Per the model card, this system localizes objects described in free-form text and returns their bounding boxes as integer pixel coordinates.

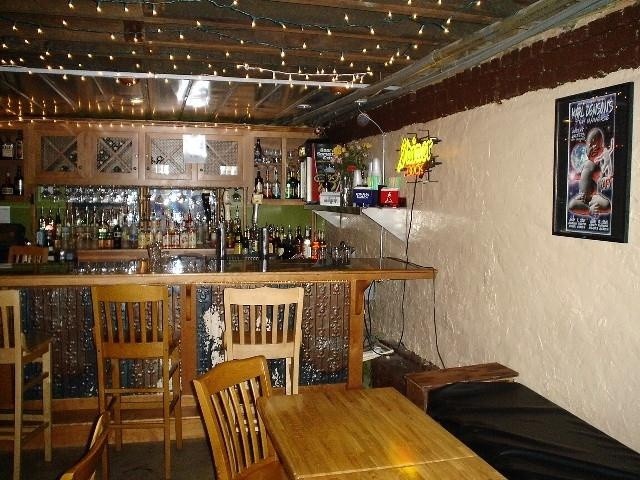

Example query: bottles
[225,210,351,269]
[322,174,332,193]
[339,168,353,208]
[0,131,23,196]
[97,137,125,173]
[48,240,77,273]
[37,206,219,249]
[57,150,77,172]
[254,138,301,199]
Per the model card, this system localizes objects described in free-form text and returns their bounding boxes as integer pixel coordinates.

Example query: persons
[569,126,611,217]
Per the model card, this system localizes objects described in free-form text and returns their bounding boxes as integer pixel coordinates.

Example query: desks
[256,386,508,480]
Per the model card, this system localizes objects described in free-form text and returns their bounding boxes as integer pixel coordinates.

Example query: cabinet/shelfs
[250,127,320,207]
[0,128,29,204]
[139,131,244,188]
[31,125,139,188]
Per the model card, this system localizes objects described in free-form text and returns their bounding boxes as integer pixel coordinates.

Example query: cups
[353,158,382,190]
[78,255,219,275]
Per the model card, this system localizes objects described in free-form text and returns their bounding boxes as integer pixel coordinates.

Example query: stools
[405,362,518,413]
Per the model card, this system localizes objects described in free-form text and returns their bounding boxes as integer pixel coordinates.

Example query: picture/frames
[552,80,635,244]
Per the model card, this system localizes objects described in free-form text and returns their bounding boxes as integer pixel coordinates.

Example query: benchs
[424,382,623,479]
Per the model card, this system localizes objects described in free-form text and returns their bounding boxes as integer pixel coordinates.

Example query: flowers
[330,138,373,171]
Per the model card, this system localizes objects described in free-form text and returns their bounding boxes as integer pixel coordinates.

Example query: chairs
[8,245,49,265]
[91,283,183,479]
[0,289,54,479]
[192,356,291,480]
[223,286,305,434]
[59,411,111,480]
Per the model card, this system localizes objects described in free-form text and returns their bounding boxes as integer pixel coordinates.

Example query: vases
[353,171,363,189]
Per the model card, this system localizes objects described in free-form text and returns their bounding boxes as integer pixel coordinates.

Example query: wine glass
[40,184,217,205]
